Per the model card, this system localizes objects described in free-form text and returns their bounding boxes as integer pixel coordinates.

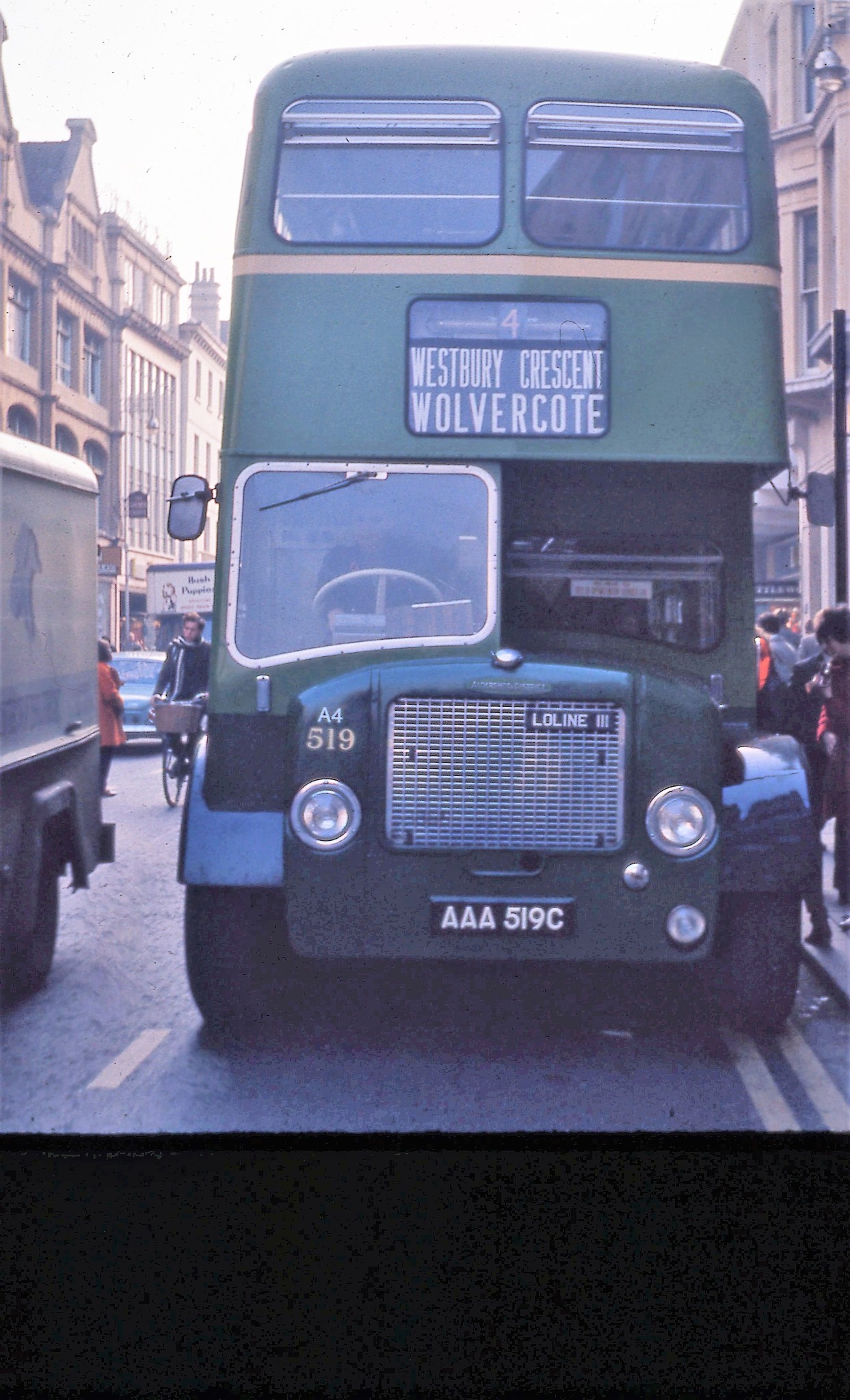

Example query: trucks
[146,562,216,653]
[0,432,116,1013]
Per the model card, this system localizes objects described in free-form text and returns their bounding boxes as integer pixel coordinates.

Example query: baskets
[154,702,201,734]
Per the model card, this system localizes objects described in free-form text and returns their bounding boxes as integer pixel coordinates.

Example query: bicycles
[153,693,206,806]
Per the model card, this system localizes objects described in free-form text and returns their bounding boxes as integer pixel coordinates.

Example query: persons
[154,612,212,776]
[117,615,178,651]
[756,601,850,943]
[95,639,124,795]
[314,500,465,626]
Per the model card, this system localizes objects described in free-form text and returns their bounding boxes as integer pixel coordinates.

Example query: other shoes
[838,916,850,929]
[805,927,831,946]
[100,789,116,796]
[169,758,185,777]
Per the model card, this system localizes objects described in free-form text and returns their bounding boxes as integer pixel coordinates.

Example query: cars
[108,651,168,747]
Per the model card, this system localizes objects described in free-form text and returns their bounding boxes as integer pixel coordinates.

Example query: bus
[162,46,812,1041]
